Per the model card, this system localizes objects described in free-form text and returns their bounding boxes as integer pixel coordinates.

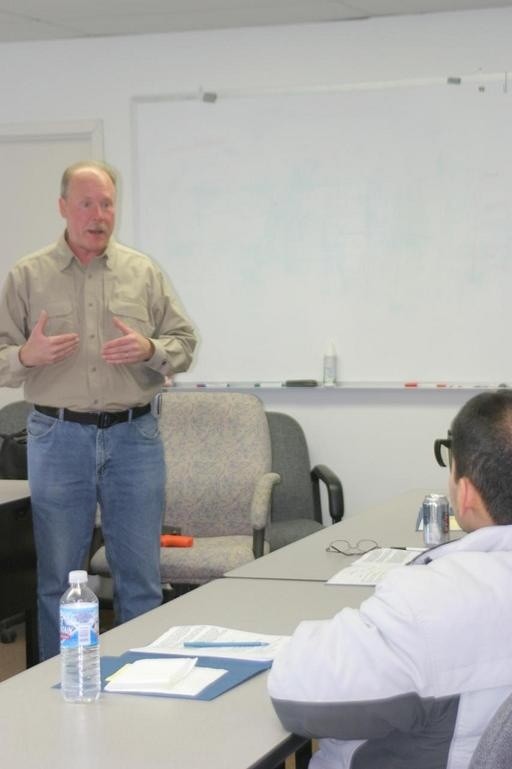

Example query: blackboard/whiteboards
[117,72,512,391]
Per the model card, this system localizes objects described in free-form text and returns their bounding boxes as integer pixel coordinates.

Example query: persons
[1,160,197,663]
[265,386,512,768]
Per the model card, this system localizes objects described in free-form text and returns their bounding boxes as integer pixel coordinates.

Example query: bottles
[59,568,104,702]
[323,341,336,388]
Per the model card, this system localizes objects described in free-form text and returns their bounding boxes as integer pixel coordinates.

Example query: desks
[225,487,471,580]
[2,576,382,768]
[0,479,34,509]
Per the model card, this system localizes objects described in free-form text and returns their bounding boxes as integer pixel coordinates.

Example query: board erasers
[285,379,318,387]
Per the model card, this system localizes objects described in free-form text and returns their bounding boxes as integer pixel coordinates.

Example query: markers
[404,382,507,388]
[172,382,262,388]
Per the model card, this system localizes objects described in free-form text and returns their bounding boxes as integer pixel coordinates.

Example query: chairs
[1,399,44,638]
[87,389,284,604]
[264,412,344,551]
[470,693,512,769]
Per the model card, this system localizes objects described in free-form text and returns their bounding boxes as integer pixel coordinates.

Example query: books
[325,547,422,588]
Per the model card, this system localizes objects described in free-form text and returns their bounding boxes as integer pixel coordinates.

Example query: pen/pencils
[184,641,268,648]
[391,546,430,551]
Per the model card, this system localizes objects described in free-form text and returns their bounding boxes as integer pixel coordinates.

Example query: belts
[32,402,153,430]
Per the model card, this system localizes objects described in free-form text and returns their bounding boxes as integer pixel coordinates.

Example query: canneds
[423,493,450,547]
